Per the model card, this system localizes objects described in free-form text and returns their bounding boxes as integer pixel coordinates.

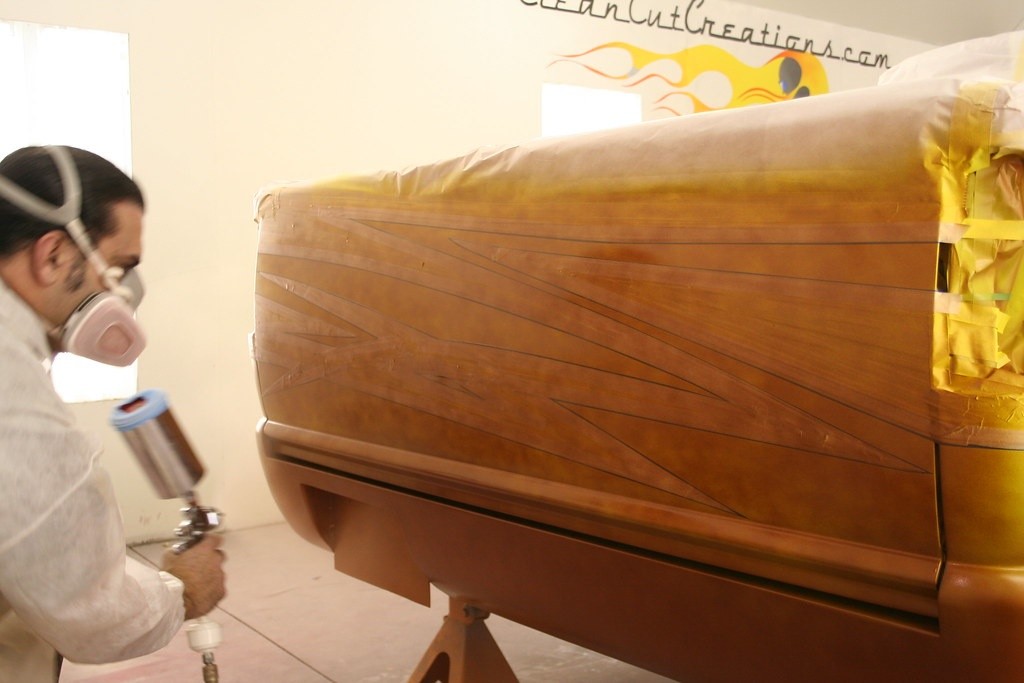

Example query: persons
[0,145,226,683]
